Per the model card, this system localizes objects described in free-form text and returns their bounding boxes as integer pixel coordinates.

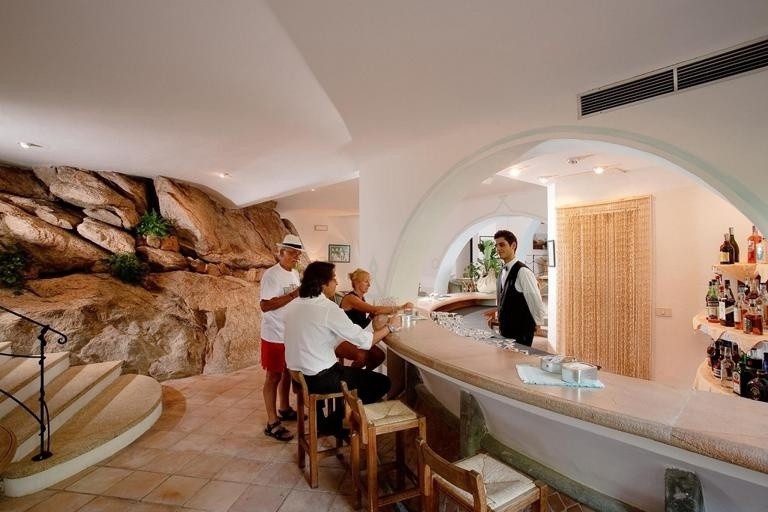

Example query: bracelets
[392,305,395,313]
[384,322,396,333]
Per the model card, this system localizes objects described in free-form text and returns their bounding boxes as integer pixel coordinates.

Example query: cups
[400,315,410,328]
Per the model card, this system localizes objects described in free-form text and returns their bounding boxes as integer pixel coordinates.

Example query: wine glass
[424,287,438,307]
[431,312,464,335]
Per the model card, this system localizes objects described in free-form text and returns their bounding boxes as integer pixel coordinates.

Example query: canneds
[402,314,411,328]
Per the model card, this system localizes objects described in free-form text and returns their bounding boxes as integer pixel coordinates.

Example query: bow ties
[499,264,508,273]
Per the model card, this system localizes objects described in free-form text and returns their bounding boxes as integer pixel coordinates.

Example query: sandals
[264,421,295,442]
[277,406,308,421]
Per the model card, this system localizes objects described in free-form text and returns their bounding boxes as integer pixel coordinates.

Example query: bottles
[746,226,767,264]
[704,274,767,333]
[718,232,732,264]
[724,226,738,262]
[704,338,767,402]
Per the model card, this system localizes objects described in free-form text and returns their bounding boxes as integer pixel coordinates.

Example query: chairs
[289,369,358,489]
[340,381,427,512]
[415,436,549,512]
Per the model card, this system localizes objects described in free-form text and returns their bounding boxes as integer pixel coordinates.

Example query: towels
[516,363,605,389]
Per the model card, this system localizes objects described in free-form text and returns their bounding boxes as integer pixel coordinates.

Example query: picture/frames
[328,244,351,263]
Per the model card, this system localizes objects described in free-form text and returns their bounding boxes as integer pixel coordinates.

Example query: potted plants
[463,239,504,293]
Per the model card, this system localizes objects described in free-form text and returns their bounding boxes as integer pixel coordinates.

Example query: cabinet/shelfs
[693,262,768,396]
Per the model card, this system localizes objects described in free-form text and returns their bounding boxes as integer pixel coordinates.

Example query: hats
[275,234,305,253]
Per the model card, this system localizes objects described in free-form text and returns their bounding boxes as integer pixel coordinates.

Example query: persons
[492,229,545,348]
[255,234,310,444]
[333,268,414,370]
[281,261,400,440]
